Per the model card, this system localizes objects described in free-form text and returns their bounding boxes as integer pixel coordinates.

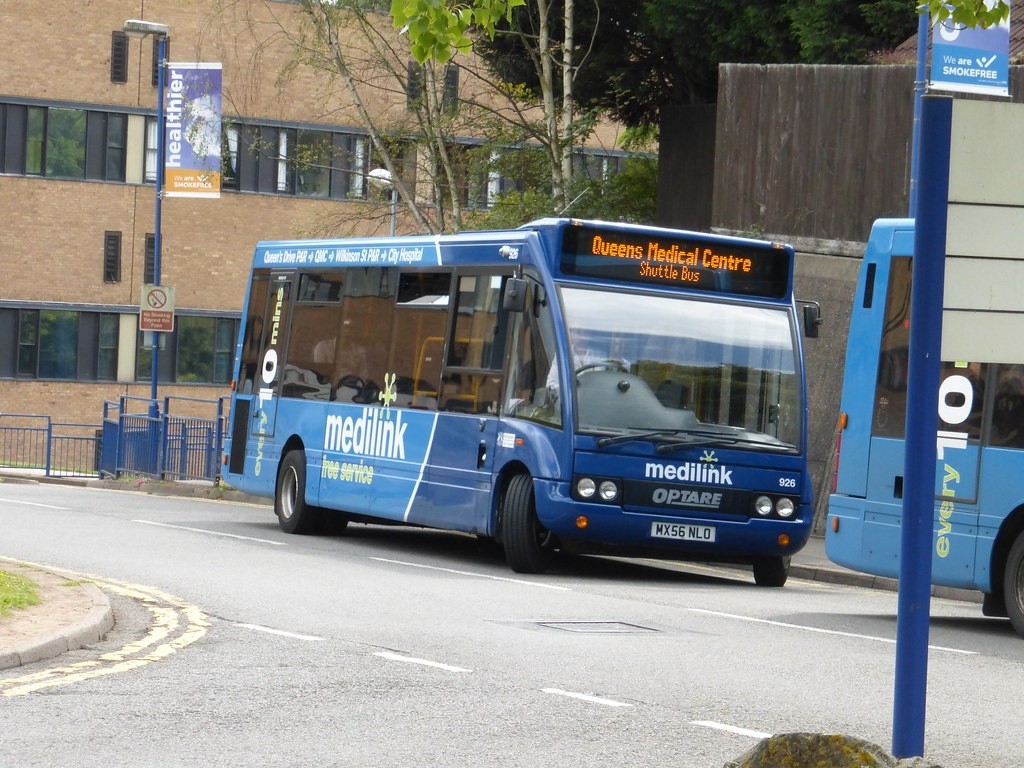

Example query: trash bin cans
[94,428,154,473]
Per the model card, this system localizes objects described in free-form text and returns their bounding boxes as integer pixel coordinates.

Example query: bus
[824,216,1024,639]
[222,215,817,588]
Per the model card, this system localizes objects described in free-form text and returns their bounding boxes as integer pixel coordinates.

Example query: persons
[313,318,369,381]
[545,314,607,389]
